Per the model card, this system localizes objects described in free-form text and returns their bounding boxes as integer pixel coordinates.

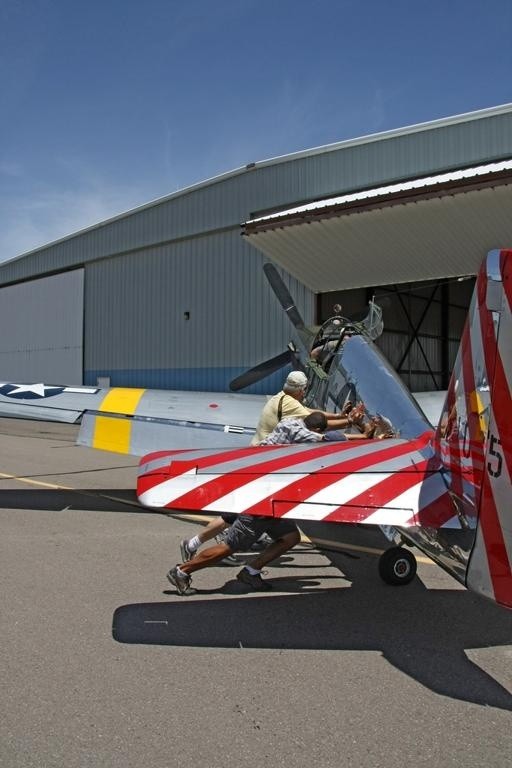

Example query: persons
[177,369,366,567]
[308,323,351,364]
[164,411,396,596]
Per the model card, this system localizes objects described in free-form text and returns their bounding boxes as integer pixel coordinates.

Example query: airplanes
[131,242,512,614]
[0,257,454,593]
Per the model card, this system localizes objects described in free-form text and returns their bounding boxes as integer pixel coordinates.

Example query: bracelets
[346,417,353,426]
[334,412,341,419]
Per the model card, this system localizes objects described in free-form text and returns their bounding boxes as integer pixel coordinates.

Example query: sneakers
[236,567,273,592]
[178,539,197,566]
[214,526,271,565]
[165,563,197,596]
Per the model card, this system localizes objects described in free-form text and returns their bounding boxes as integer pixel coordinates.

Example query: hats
[339,326,355,336]
[286,370,312,386]
